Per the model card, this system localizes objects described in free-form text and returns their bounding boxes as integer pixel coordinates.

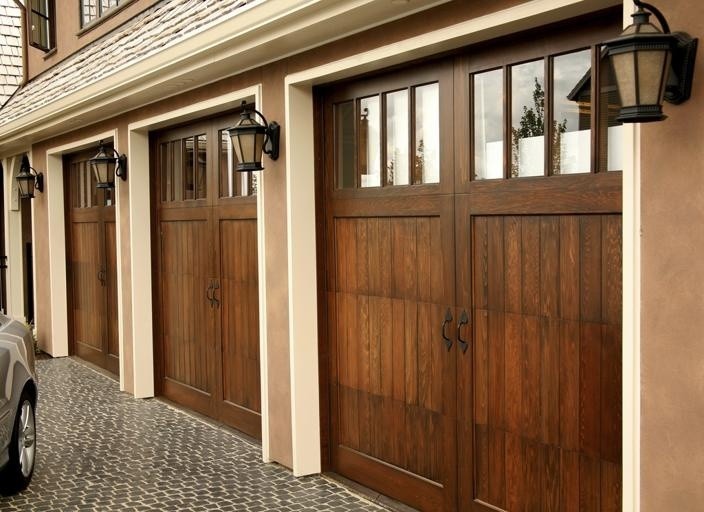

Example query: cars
[0,312,39,489]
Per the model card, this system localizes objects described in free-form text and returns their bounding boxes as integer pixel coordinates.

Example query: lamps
[227,101,281,176]
[601,1,698,127]
[87,140,127,189]
[15,154,44,201]
